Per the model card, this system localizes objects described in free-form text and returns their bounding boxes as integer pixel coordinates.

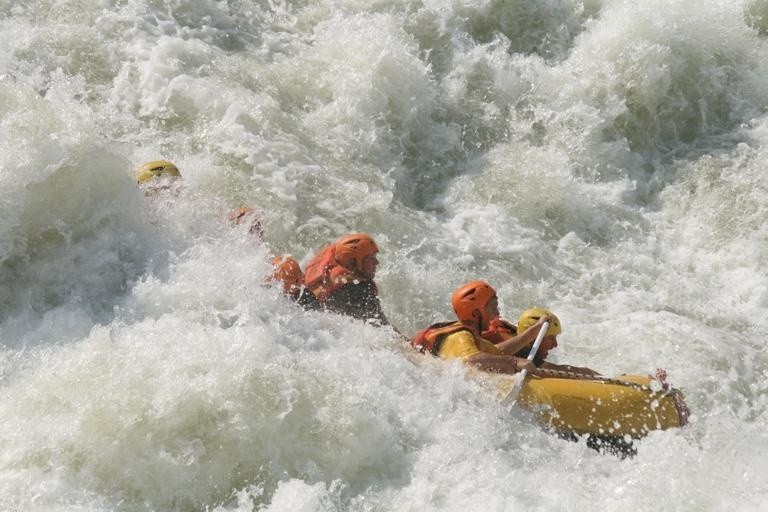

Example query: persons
[410,281,552,376]
[481,308,603,377]
[272,258,321,312]
[309,232,389,324]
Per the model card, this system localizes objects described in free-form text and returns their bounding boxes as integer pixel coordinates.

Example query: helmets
[227,207,263,235]
[272,257,305,293]
[452,283,497,327]
[515,309,560,338]
[335,235,378,274]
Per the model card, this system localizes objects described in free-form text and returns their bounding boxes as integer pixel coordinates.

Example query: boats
[458,362,694,461]
